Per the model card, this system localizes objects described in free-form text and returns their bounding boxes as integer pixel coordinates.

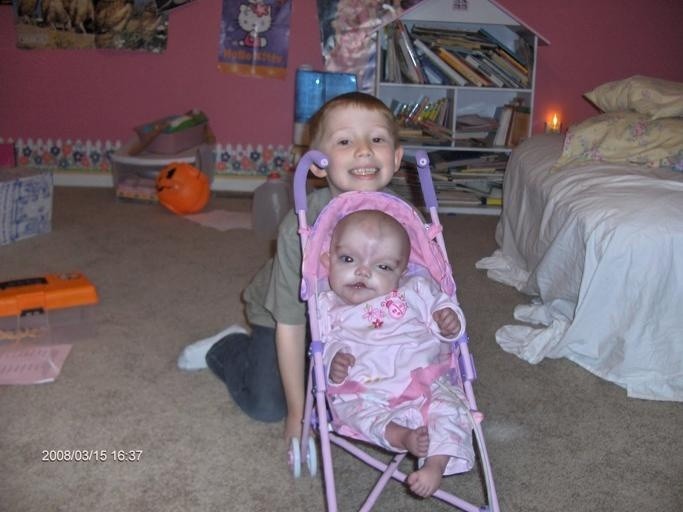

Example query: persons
[317,209,476,500]
[177,90,427,446]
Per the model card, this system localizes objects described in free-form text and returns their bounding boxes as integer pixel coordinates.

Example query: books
[385,19,534,89]
[391,149,509,207]
[389,94,452,147]
[455,96,531,148]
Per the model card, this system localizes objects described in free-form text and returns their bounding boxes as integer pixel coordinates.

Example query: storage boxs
[106,149,196,205]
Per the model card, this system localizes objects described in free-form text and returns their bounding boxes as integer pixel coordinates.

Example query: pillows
[583,74,683,120]
[553,111,683,173]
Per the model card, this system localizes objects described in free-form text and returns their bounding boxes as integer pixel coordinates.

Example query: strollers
[285,147,501,512]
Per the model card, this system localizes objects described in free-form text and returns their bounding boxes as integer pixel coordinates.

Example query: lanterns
[155,161,211,215]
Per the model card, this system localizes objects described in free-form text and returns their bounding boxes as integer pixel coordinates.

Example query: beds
[475,131,683,403]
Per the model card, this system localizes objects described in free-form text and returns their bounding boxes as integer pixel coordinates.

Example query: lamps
[552,114,558,132]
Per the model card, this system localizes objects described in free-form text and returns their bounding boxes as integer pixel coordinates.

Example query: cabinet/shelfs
[365,0,551,216]
